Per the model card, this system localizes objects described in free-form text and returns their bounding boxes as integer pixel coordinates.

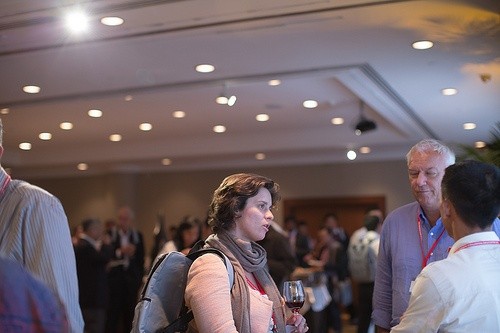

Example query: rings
[304,324,309,330]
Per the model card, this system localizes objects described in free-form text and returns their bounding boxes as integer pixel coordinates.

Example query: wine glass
[283,281,306,326]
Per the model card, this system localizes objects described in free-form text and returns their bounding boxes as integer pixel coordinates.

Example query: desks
[290,269,327,333]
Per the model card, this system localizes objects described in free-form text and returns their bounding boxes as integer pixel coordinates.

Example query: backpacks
[130,240,234,333]
[348,239,378,283]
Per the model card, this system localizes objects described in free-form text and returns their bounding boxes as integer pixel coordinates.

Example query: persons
[390,159,500,333]
[0,119,211,333]
[185,174,310,333]
[371,139,500,333]
[256,208,385,333]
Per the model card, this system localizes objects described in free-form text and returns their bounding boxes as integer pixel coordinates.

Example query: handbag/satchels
[335,281,353,306]
[310,284,332,312]
